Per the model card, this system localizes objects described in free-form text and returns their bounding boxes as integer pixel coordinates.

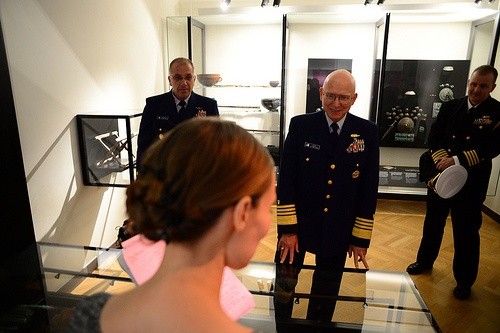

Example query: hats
[428,164,468,199]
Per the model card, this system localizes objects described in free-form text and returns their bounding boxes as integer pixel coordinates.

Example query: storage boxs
[76,108,143,188]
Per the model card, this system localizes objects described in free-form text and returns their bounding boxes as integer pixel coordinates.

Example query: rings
[281,247,284,250]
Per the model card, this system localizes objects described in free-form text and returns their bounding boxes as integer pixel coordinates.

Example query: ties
[468,106,476,115]
[330,122,340,143]
[178,100,186,114]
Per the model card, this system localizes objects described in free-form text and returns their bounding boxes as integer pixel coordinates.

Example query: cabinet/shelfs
[197,85,281,135]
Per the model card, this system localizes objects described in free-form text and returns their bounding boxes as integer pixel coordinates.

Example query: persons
[406,65,500,300]
[48,116,277,333]
[272,68,380,333]
[306,78,322,113]
[135,57,220,177]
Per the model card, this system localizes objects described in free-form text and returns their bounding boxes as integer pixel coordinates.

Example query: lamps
[260,0,269,7]
[220,0,232,11]
[377,0,385,6]
[273,0,280,7]
[488,0,496,5]
[474,0,482,6]
[363,0,374,6]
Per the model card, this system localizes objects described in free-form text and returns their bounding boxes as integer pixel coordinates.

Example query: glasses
[170,74,196,81]
[322,87,355,104]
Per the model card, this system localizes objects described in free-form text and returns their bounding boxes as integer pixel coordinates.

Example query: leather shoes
[453,286,471,300]
[407,262,428,275]
